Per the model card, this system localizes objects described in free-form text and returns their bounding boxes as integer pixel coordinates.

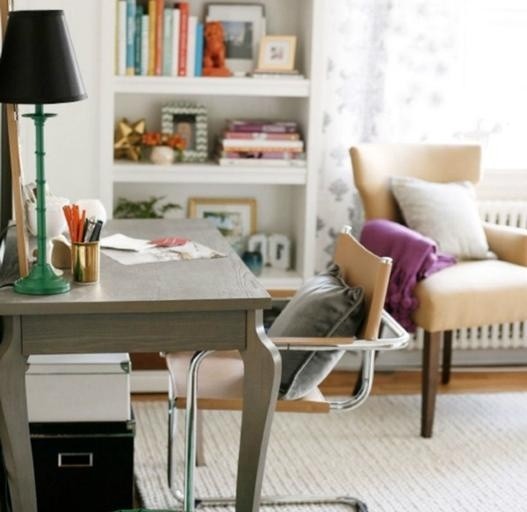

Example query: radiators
[400,205,526,354]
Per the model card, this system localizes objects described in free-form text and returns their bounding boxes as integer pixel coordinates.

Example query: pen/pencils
[101,247,139,252]
[63,203,102,242]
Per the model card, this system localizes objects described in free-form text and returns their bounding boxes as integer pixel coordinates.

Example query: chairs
[349,143,527,437]
[165,225,409,512]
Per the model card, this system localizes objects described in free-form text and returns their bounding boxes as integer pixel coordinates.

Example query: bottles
[75,198,107,230]
[25,196,71,239]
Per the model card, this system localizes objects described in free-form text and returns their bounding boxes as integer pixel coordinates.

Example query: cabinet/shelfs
[103,0,318,298]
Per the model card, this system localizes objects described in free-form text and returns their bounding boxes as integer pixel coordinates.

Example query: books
[213,117,305,165]
[114,1,207,78]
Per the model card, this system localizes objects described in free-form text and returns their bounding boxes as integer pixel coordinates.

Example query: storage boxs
[26,353,132,424]
[31,420,133,512]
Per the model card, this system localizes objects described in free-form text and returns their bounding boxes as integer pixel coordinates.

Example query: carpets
[134,391,527,512]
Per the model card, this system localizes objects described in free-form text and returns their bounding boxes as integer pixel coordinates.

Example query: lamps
[1,10,90,298]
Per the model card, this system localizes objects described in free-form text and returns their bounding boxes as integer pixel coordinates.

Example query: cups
[71,242,100,284]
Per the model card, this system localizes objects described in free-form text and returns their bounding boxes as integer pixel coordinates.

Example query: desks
[0,218,283,512]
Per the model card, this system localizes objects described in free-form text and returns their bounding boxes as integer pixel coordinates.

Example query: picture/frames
[186,198,257,252]
[257,34,298,71]
[161,100,210,163]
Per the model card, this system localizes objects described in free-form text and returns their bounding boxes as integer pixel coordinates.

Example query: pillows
[390,170,497,261]
[268,263,366,401]
